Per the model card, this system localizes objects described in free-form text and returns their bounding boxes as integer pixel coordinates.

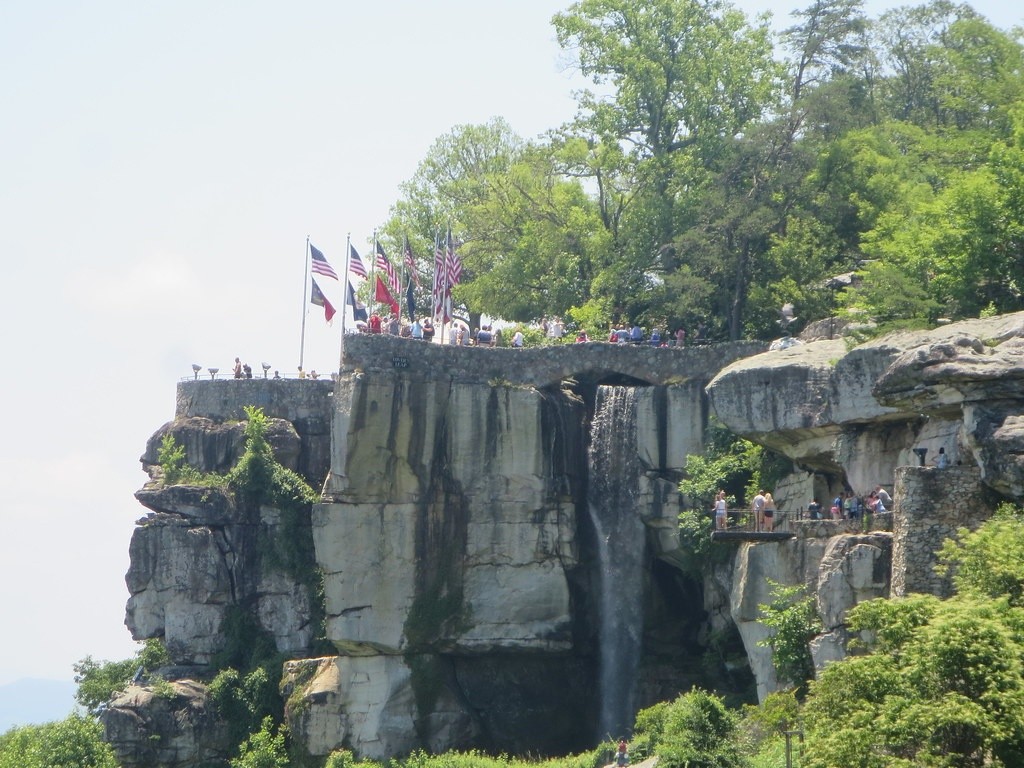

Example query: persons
[694,324,708,344]
[808,485,891,519]
[529,316,565,340]
[273,371,281,379]
[233,357,252,379]
[298,366,305,378]
[614,742,629,768]
[578,330,592,342]
[311,371,317,378]
[450,323,503,347]
[609,324,684,347]
[510,328,523,347]
[931,447,950,468]
[357,311,435,340]
[711,492,727,530]
[751,490,774,532]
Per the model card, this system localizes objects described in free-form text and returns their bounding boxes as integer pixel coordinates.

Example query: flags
[310,244,340,281]
[346,228,461,324]
[311,276,335,322]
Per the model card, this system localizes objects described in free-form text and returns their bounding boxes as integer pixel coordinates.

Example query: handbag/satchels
[610,335,618,341]
[817,512,823,519]
[831,507,839,514]
